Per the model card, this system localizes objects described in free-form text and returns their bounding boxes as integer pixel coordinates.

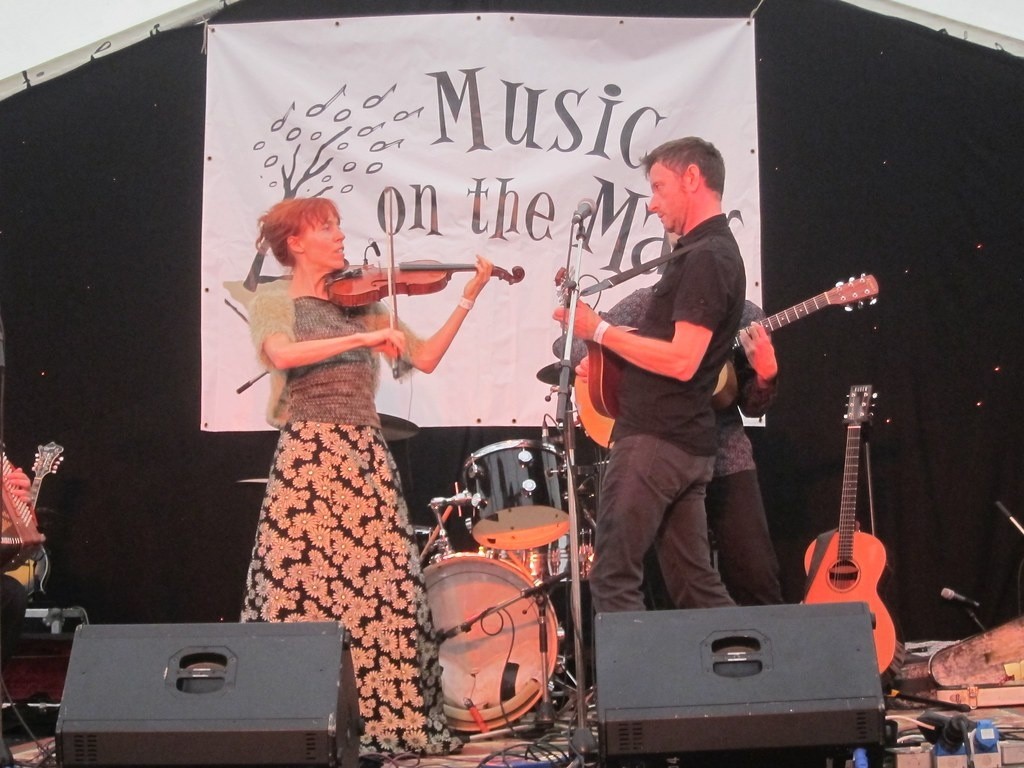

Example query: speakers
[54,621,367,767]
[589,600,885,768]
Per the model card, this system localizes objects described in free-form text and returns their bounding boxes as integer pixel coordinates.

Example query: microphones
[541,420,550,444]
[571,197,597,224]
[940,588,980,607]
[937,714,967,754]
[362,242,381,266]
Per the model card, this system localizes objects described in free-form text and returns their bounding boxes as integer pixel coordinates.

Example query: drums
[463,438,569,550]
[478,464,596,583]
[422,553,558,731]
[415,526,452,570]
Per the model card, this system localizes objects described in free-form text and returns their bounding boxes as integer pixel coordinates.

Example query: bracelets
[458,296,475,311]
[593,319,611,345]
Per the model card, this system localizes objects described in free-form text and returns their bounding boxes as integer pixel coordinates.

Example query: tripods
[433,568,596,744]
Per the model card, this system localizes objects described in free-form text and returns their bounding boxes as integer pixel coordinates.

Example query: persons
[552,137,747,617]
[237,197,494,759]
[587,285,784,611]
[0,441,33,675]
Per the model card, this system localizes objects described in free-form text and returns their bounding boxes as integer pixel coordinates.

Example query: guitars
[574,273,879,451]
[555,265,639,420]
[799,384,906,687]
[4,441,63,598]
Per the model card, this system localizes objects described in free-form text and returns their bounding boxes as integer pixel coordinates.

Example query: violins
[327,259,526,307]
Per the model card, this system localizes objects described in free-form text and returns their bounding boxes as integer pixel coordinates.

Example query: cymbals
[535,360,581,390]
[376,412,420,441]
[235,479,269,484]
